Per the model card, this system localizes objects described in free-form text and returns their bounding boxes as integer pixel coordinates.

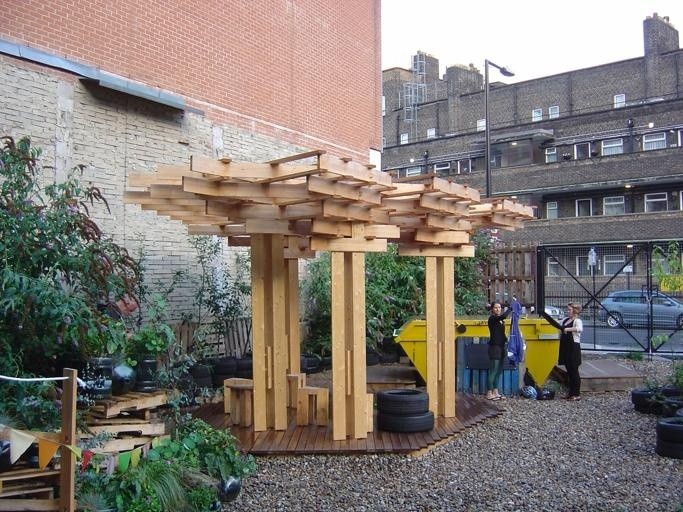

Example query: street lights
[421,149,430,175]
[482,57,514,198]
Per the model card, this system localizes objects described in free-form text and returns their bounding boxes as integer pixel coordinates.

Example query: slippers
[486,396,507,400]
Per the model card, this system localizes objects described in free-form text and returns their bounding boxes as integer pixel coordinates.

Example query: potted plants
[124,325,163,394]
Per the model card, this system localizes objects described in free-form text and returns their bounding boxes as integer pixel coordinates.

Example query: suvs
[598,290,682,329]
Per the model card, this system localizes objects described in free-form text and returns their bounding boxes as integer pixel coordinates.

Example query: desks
[89,386,180,421]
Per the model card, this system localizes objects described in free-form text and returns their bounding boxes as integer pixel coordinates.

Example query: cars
[476,291,564,321]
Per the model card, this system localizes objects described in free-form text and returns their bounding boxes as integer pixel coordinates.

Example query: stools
[223,373,331,433]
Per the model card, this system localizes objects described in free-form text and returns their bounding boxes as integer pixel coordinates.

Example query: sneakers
[566,395,581,401]
[560,393,574,399]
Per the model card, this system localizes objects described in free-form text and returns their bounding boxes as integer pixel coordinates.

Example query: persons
[103,294,137,342]
[558,302,583,401]
[486,295,517,401]
